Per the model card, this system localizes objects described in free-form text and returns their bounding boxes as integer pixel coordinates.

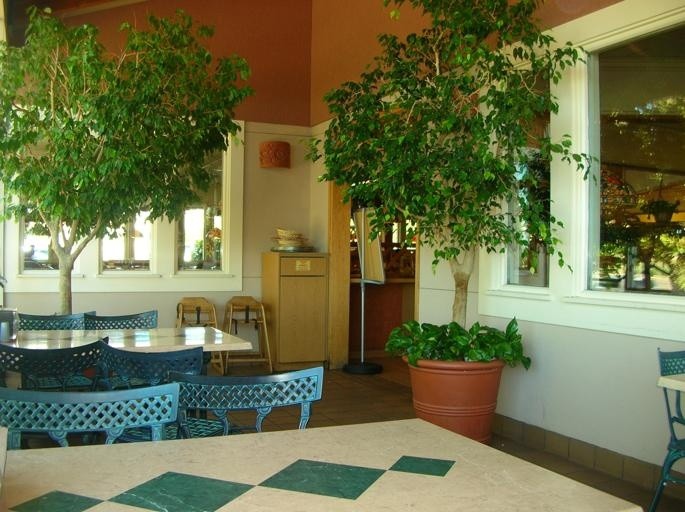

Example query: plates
[271,246,315,252]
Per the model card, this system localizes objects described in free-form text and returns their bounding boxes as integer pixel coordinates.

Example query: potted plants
[300,0,640,444]
[643,200,681,223]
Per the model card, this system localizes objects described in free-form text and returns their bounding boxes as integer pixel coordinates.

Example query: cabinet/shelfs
[263,250,328,370]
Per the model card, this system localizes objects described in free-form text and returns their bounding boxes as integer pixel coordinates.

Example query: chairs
[17,312,96,389]
[1,338,108,391]
[649,347,684,512]
[170,366,324,438]
[0,382,180,451]
[98,338,231,441]
[223,295,273,374]
[176,296,225,374]
[83,310,158,389]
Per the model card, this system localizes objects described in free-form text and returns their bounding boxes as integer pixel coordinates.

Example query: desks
[18,327,253,352]
[1,416,644,512]
[658,374,685,391]
